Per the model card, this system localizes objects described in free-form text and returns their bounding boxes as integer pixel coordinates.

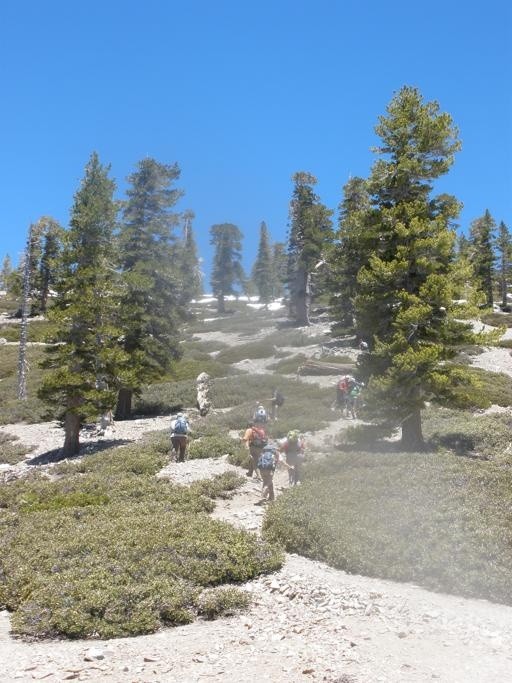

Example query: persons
[170,412,192,462]
[265,383,279,419]
[336,375,350,415]
[345,377,364,418]
[252,401,268,423]
[239,422,306,499]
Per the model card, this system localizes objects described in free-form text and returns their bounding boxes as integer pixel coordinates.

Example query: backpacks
[287,431,300,450]
[251,429,267,447]
[258,452,273,471]
[255,410,266,422]
[176,418,188,434]
[277,394,283,404]
